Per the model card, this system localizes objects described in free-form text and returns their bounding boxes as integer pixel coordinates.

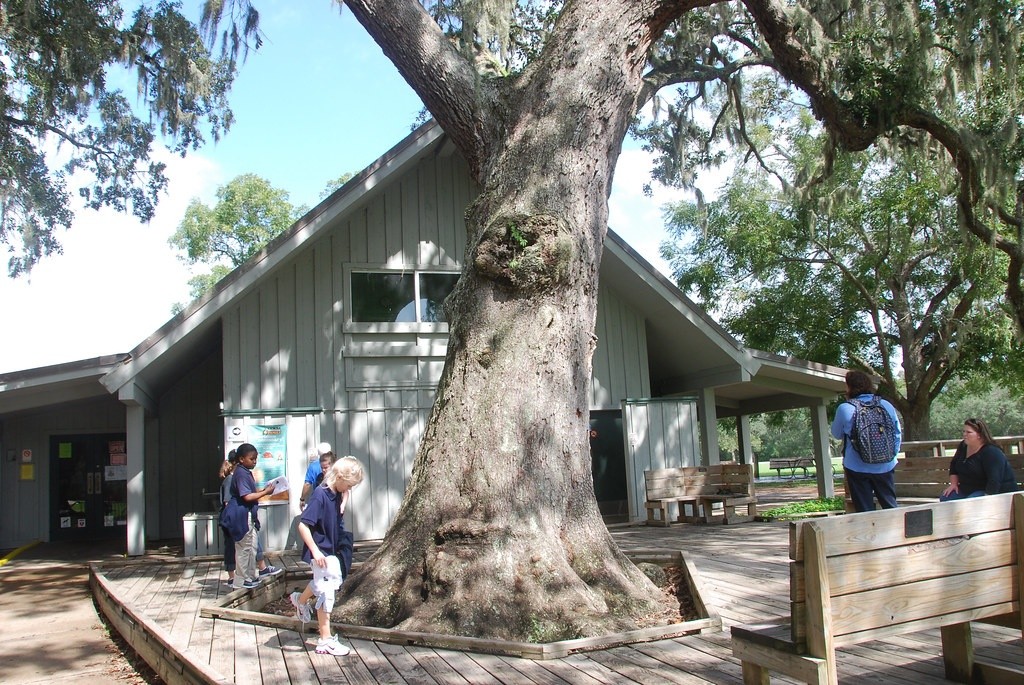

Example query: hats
[317,442,332,456]
[227,448,238,460]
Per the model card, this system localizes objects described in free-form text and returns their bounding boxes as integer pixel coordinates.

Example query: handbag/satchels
[218,496,249,542]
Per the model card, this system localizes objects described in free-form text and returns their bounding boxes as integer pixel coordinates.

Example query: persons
[831,371,904,513]
[940,418,1018,502]
[290,442,366,656]
[218,443,282,588]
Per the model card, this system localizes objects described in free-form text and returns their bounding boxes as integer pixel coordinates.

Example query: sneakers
[315,633,351,656]
[290,591,313,624]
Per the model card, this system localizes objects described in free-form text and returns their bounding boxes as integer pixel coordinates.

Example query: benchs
[644,435,1024,685]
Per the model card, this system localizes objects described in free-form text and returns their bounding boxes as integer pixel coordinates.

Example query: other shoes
[258,565,283,577]
[232,577,263,589]
[227,577,234,588]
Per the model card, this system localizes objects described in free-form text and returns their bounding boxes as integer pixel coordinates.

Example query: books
[266,475,289,497]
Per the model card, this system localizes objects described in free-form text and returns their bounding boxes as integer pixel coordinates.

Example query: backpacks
[842,395,896,464]
